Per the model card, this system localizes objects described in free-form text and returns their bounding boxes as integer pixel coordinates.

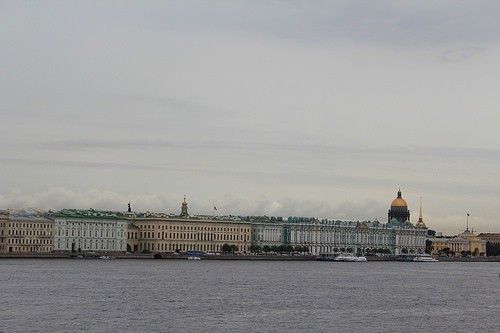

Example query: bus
[187,250,202,255]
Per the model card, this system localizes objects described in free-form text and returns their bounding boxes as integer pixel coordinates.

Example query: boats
[413,254,438,261]
[316,253,338,261]
[333,253,367,262]
